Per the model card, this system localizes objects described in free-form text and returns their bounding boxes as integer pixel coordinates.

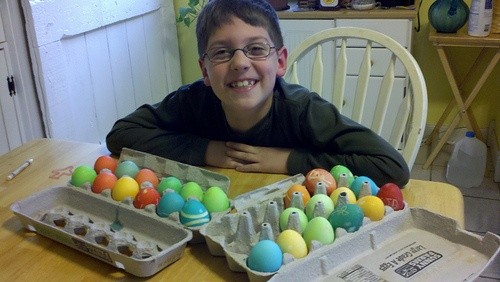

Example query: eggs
[247,164,404,273]
[71,154,229,227]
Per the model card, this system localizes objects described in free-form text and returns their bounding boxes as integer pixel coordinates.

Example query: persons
[106,0,410,189]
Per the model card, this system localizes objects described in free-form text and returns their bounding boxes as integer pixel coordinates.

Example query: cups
[345,0,381,10]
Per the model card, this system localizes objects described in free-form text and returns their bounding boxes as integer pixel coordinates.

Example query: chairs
[281,27,428,175]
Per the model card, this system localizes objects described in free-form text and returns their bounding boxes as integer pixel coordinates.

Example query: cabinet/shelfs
[277,19,414,150]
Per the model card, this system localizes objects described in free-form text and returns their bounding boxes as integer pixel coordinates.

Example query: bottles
[446,131,487,188]
[468,0,493,37]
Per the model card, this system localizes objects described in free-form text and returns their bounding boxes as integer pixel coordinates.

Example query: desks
[422,24,500,170]
[0,137,465,282]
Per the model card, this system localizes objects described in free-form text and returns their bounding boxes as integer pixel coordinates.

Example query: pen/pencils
[7,159,33,181]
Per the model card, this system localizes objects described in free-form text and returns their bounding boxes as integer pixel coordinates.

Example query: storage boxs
[198,173,500,282]
[10,148,230,278]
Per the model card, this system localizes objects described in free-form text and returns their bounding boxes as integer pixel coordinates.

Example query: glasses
[200,40,278,64]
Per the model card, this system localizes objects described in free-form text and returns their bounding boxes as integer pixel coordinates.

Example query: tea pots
[428,0,469,33]
